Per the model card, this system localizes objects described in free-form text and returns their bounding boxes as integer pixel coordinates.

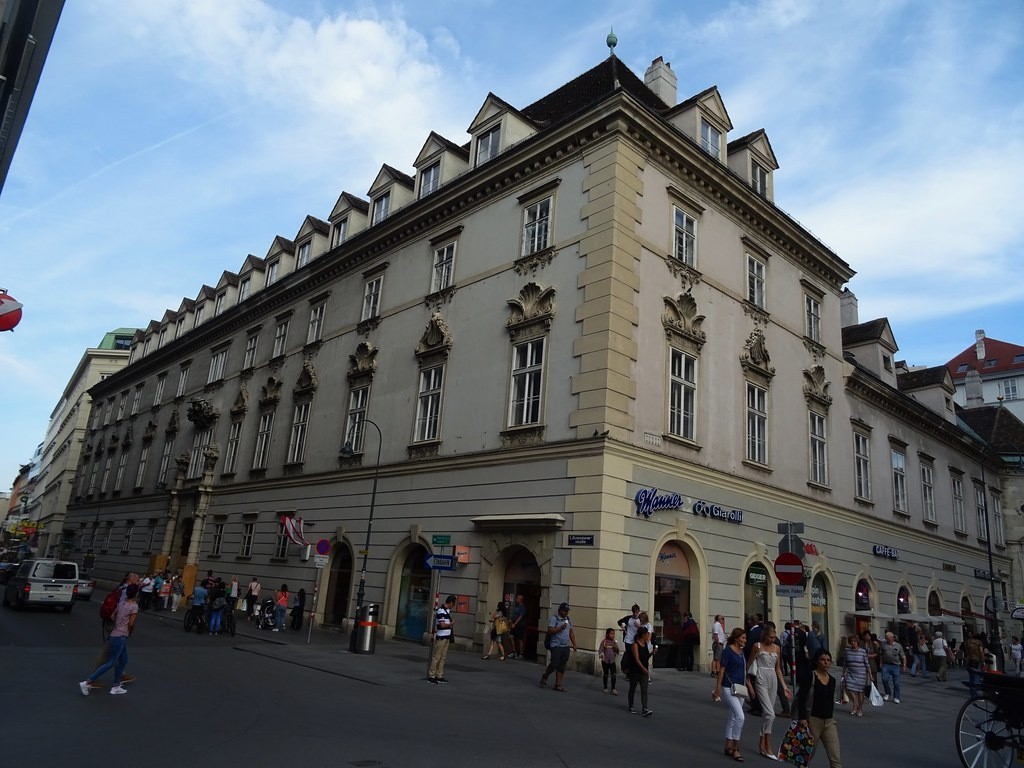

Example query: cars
[76,571,96,601]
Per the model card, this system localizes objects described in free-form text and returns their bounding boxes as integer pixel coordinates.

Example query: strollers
[255,596,275,630]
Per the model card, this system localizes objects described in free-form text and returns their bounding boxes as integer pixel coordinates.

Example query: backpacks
[100,585,128,620]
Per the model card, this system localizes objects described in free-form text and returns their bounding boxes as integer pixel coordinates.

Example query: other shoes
[110,686,127,694]
[272,628,279,632]
[79,680,92,696]
[121,673,136,683]
[427,651,982,763]
[88,681,105,689]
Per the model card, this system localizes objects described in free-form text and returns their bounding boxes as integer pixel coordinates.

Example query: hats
[559,603,571,611]
[935,631,942,637]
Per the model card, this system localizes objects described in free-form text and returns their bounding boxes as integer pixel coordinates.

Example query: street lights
[980,441,1024,673]
[26,500,43,556]
[80,487,104,572]
[337,419,382,653]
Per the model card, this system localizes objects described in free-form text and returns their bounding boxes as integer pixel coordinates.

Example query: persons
[79,571,140,694]
[910,627,951,681]
[290,588,305,630]
[483,595,525,661]
[247,576,261,623]
[840,630,907,716]
[678,612,699,672]
[708,613,841,768]
[540,602,576,692]
[271,582,289,631]
[598,626,620,694]
[427,595,457,684]
[188,569,240,637]
[958,628,1024,702]
[137,570,184,612]
[616,601,657,716]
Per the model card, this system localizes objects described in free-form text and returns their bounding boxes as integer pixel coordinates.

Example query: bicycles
[184,597,236,637]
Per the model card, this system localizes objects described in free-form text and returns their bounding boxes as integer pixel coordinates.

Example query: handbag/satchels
[917,635,930,653]
[449,628,455,643]
[213,596,227,609]
[835,681,849,705]
[731,683,749,697]
[868,681,885,707]
[245,583,252,599]
[776,720,814,768]
[237,582,241,596]
[237,599,248,610]
[747,642,758,676]
[495,612,511,635]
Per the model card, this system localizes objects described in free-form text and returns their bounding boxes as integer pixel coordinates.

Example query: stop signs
[774,552,803,584]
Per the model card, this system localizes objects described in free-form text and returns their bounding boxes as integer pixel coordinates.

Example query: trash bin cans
[349,601,383,656]
[969,653,999,702]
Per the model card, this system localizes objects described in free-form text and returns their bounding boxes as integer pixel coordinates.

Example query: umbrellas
[848,608,891,633]
[892,611,931,625]
[928,614,965,639]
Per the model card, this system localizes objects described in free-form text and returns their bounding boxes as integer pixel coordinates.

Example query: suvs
[4,559,80,612]
